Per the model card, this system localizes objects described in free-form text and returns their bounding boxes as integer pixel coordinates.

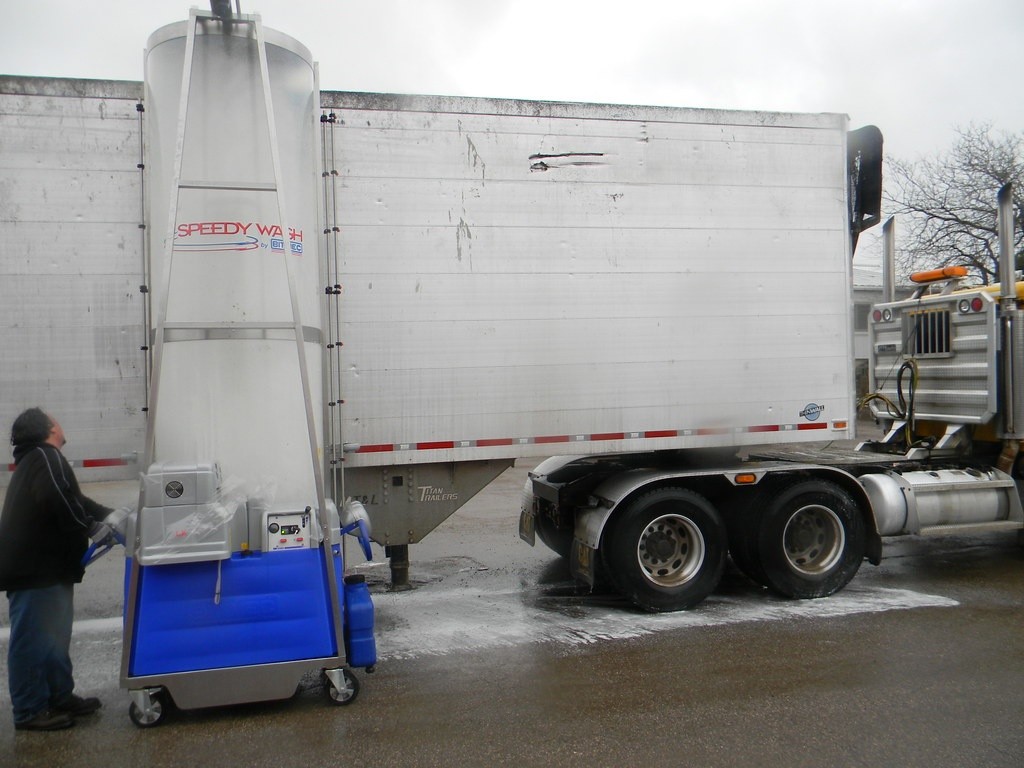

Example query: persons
[0,408,117,731]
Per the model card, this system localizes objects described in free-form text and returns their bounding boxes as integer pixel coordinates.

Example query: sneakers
[51,697,102,716]
[14,709,74,731]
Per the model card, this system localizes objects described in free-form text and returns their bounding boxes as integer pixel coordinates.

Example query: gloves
[88,522,116,547]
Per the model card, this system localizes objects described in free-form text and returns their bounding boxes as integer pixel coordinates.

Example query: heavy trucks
[511,180,1024,614]
